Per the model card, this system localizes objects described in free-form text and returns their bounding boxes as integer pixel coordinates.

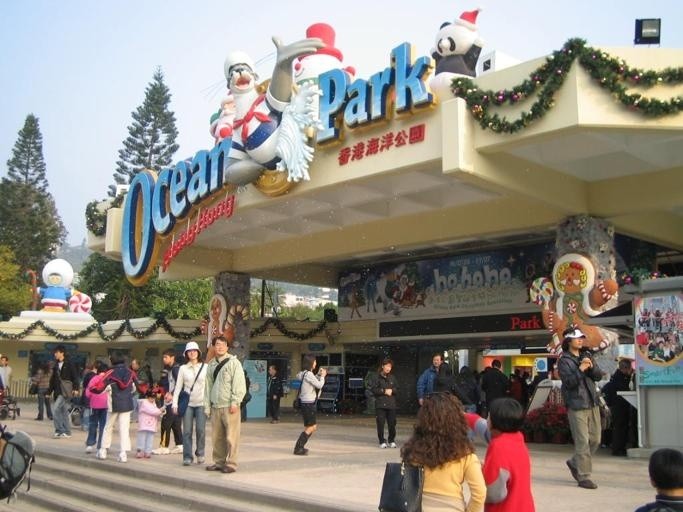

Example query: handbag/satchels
[291,370,311,410]
[27,376,40,395]
[377,451,425,512]
[55,367,76,400]
[177,363,204,417]
[212,359,253,409]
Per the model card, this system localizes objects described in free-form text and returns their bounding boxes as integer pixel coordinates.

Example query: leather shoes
[222,465,236,473]
[566,460,579,483]
[578,480,598,489]
[206,465,221,471]
[182,458,192,466]
[197,456,205,463]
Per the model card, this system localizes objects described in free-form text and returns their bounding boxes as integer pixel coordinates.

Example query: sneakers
[379,443,387,449]
[84,446,93,453]
[145,453,151,459]
[95,449,107,460]
[135,452,143,458]
[51,433,63,439]
[116,453,127,463]
[389,442,396,448]
[63,433,72,439]
[152,447,170,455]
[169,444,184,455]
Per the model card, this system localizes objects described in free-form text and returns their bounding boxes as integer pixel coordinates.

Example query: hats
[181,342,203,358]
[564,329,587,341]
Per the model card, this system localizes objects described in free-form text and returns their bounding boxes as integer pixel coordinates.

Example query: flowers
[546,408,569,431]
[526,409,546,431]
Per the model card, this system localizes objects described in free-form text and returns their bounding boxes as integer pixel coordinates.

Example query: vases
[533,431,547,443]
[552,431,568,444]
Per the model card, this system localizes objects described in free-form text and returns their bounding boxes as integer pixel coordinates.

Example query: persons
[372,359,400,449]
[558,327,603,489]
[204,336,246,472]
[136,388,165,459]
[435,362,453,392]
[240,370,252,423]
[514,369,520,381]
[401,392,487,512]
[601,358,633,457]
[172,342,209,466]
[481,359,512,397]
[636,303,683,363]
[32,361,54,421]
[151,348,185,454]
[522,372,533,403]
[266,365,284,424]
[293,354,327,455]
[417,354,445,405]
[633,448,683,512]
[510,375,521,399]
[85,364,108,454]
[96,351,145,463]
[455,366,480,404]
[1,356,11,391]
[0,420,7,466]
[131,358,148,397]
[464,398,536,512]
[50,346,79,439]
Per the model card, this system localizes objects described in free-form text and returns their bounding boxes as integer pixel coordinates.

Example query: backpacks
[0,431,34,504]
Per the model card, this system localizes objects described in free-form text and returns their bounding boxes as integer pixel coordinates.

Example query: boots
[294,431,311,455]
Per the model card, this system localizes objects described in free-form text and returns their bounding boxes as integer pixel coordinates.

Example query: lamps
[635,18,661,45]
